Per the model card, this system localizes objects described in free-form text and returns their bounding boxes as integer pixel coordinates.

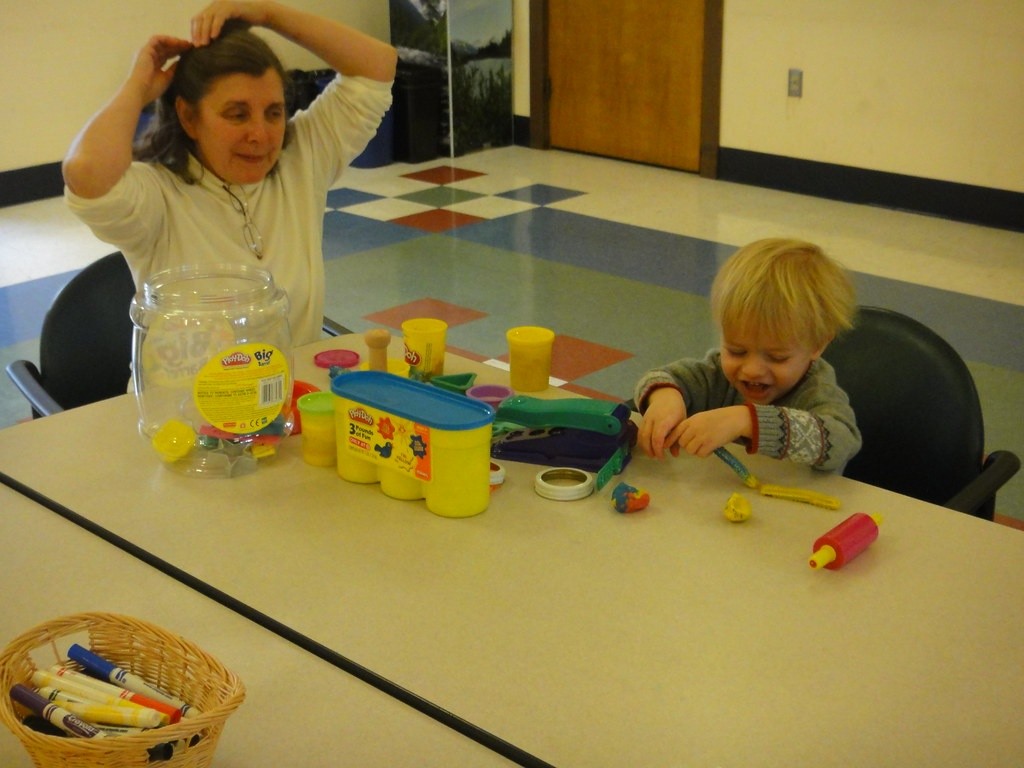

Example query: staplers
[491,394,632,475]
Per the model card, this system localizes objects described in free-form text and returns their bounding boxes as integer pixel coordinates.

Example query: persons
[62,0,396,395]
[633,236,862,476]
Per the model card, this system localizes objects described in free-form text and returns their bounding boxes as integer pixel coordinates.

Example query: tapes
[534,467,594,501]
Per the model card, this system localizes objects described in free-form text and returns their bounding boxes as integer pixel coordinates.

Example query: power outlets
[786,69,804,101]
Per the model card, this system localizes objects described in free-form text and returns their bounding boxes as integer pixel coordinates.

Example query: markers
[9,642,203,760]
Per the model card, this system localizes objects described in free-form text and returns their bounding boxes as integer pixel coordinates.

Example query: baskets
[0,611,248,768]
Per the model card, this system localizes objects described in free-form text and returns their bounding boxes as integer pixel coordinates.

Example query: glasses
[222,181,264,260]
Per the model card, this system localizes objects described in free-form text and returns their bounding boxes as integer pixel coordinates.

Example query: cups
[507,326,554,391]
[298,371,494,516]
[402,317,447,381]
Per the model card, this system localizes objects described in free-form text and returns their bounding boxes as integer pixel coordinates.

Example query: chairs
[813,307,1017,522]
[6,247,354,419]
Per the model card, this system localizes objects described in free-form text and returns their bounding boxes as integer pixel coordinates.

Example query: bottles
[129,260,297,476]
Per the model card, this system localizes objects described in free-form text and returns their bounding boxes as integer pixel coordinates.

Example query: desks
[0,388,1023,767]
